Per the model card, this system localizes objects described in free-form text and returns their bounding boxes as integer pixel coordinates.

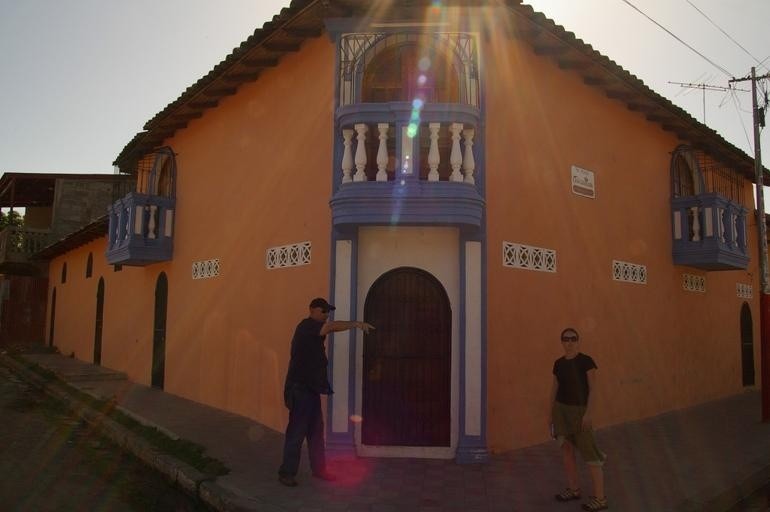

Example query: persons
[279,298,376,487]
[549,328,612,510]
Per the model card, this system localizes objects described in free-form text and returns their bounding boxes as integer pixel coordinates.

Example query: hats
[309,297,336,312]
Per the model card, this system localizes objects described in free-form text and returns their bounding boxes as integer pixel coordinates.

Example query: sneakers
[312,471,338,482]
[581,496,608,512]
[277,474,299,488]
[555,487,582,502]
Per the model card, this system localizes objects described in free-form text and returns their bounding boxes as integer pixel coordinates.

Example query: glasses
[316,307,332,314]
[561,335,579,343]
